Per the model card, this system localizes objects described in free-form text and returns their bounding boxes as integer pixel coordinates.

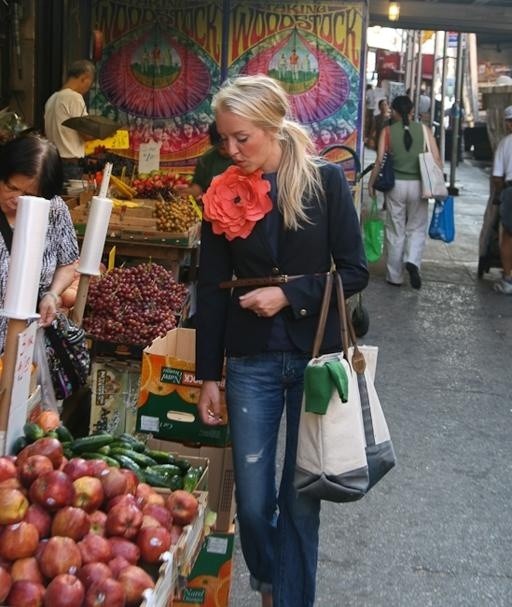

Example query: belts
[218,276,302,286]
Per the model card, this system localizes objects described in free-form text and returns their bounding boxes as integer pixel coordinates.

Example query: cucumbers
[13,423,202,490]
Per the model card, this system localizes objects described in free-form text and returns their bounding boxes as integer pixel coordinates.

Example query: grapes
[82,262,189,347]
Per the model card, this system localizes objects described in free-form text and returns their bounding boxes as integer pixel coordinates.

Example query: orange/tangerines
[131,170,194,182]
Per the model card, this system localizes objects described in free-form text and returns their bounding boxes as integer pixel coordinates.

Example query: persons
[89,90,214,154]
[488,107,512,294]
[141,43,173,74]
[44,59,99,193]
[279,49,310,81]
[365,84,431,152]
[306,98,357,154]
[0,131,80,356]
[194,73,370,607]
[370,95,442,289]
[183,121,237,212]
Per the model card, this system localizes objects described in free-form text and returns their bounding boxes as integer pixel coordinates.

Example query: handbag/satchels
[42,314,91,400]
[419,153,449,200]
[372,153,394,192]
[429,198,454,242]
[295,344,396,502]
[363,220,384,263]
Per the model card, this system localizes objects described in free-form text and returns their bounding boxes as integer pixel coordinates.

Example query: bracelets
[41,292,58,304]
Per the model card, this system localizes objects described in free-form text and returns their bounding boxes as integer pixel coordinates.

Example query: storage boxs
[169,533,234,607]
[144,433,236,532]
[136,328,234,446]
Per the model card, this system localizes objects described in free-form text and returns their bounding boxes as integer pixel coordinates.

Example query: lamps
[388,1,401,21]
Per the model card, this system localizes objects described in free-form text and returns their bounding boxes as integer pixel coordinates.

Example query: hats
[504,106,512,119]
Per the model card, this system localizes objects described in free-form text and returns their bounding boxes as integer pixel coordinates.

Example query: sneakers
[405,262,421,289]
[386,280,401,287]
[493,280,512,295]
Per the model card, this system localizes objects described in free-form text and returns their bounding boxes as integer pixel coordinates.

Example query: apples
[1,439,198,607]
[37,410,59,431]
[133,175,188,196]
